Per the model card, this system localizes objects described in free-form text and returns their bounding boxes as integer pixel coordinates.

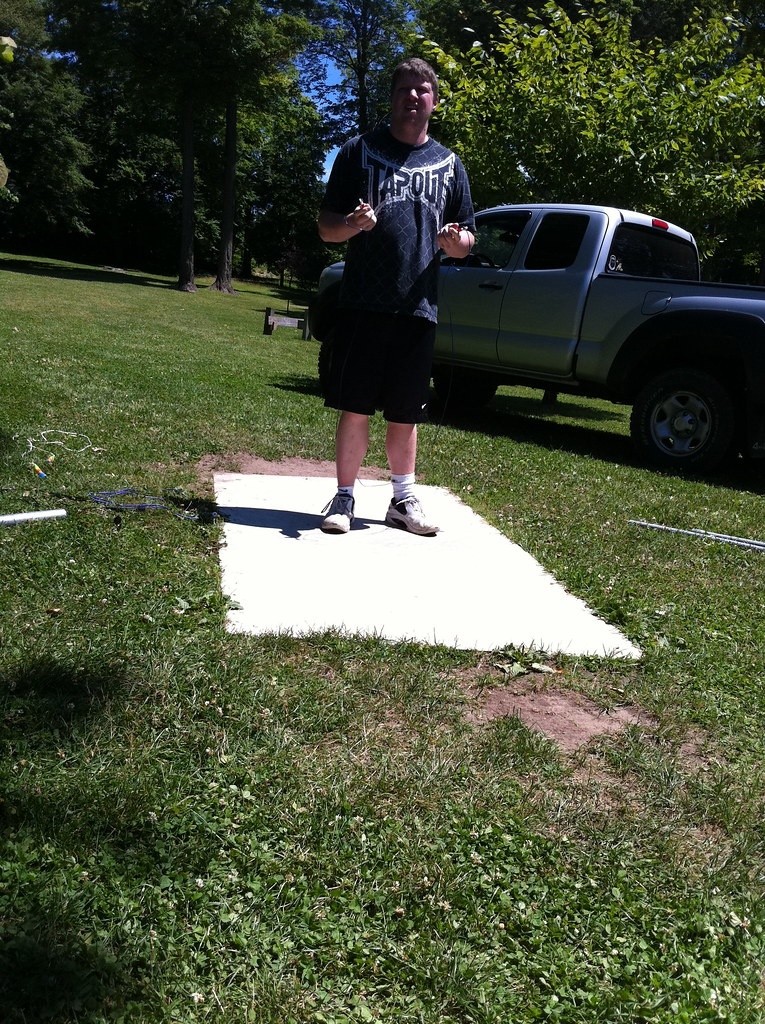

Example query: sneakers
[386,495,442,536]
[321,495,356,534]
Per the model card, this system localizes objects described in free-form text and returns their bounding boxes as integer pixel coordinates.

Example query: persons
[318,57,477,538]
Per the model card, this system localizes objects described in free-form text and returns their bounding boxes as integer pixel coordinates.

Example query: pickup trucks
[304,201,764,483]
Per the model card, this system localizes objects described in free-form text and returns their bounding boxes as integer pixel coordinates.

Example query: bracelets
[343,216,362,232]
